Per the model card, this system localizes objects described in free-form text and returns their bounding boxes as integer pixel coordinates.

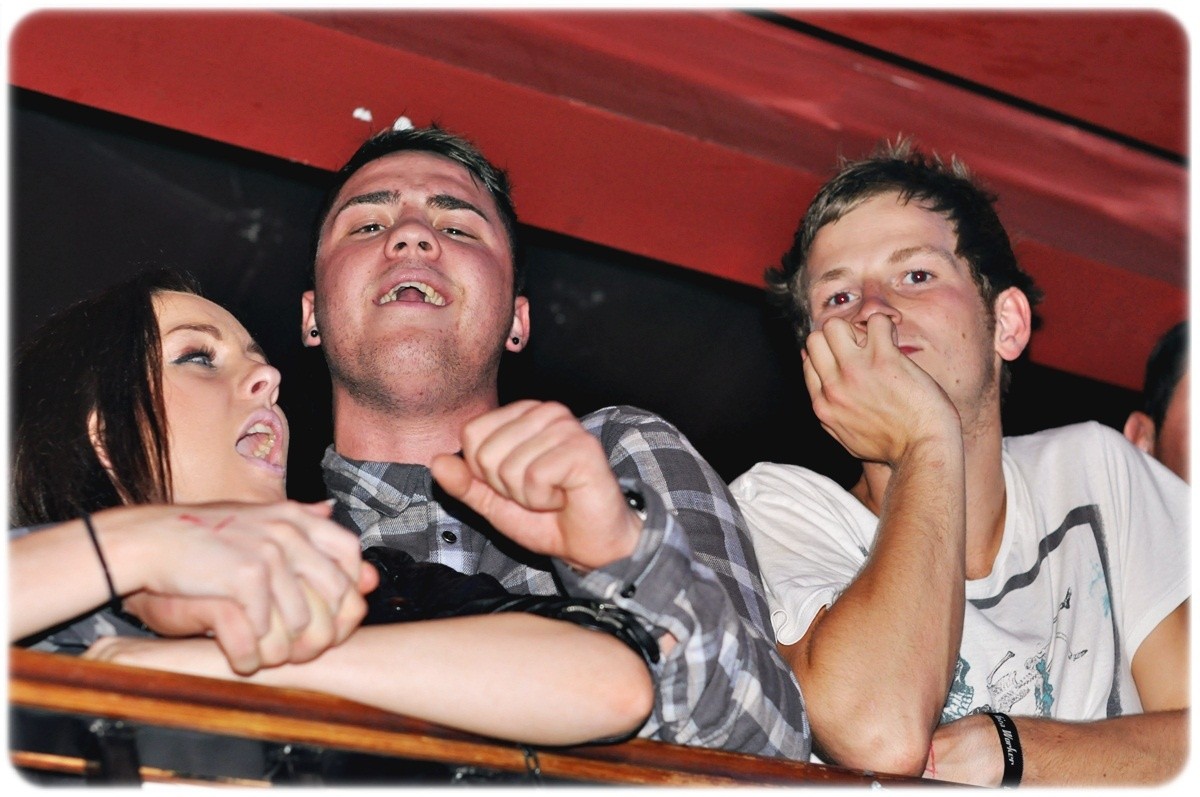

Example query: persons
[123,128,813,767]
[8,274,660,778]
[1124,319,1191,482]
[725,158,1191,787]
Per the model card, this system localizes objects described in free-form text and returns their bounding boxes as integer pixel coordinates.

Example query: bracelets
[82,514,116,601]
[985,712,1023,787]
